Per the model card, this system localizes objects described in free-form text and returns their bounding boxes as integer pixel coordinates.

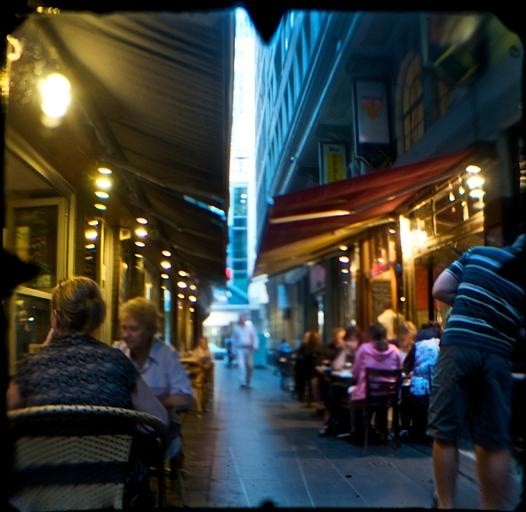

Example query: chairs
[5,406,170,511]
[348,367,402,450]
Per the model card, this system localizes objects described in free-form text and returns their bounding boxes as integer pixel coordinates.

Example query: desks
[149,386,170,399]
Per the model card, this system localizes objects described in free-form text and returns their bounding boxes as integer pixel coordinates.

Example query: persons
[278,297,444,442]
[194,336,211,359]
[231,310,259,389]
[6,276,195,426]
[426,234,526,512]
